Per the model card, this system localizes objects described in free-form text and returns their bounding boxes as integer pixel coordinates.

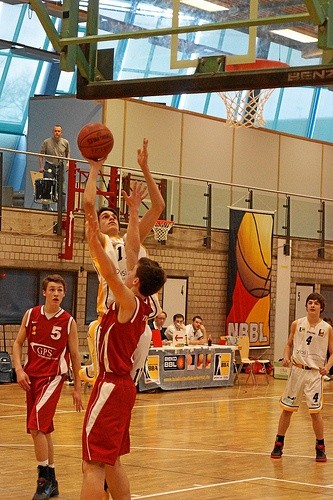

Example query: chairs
[233,336,270,385]
[30,171,52,210]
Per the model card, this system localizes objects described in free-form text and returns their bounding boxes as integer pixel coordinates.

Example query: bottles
[207,334,212,346]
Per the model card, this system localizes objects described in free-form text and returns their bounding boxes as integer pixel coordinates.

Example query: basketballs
[77,122,114,160]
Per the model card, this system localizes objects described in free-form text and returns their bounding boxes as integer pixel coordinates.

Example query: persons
[186,316,207,345]
[13,274,85,500]
[148,311,167,345]
[271,293,333,462]
[163,314,186,340]
[80,180,167,500]
[78,137,166,385]
[39,125,70,212]
[322,318,333,381]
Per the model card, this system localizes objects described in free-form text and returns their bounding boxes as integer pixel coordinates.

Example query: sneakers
[271,441,283,458]
[32,478,59,500]
[316,443,327,462]
[323,375,331,380]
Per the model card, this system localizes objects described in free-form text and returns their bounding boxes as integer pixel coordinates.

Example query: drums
[34,177,58,204]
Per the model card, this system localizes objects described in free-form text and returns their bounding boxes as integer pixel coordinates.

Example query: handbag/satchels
[234,357,273,374]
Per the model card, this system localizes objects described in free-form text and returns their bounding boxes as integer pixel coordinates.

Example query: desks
[138,344,239,387]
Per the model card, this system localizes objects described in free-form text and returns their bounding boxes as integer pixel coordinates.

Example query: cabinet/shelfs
[23,96,332,240]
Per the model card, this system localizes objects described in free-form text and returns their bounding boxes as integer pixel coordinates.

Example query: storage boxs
[273,361,291,380]
